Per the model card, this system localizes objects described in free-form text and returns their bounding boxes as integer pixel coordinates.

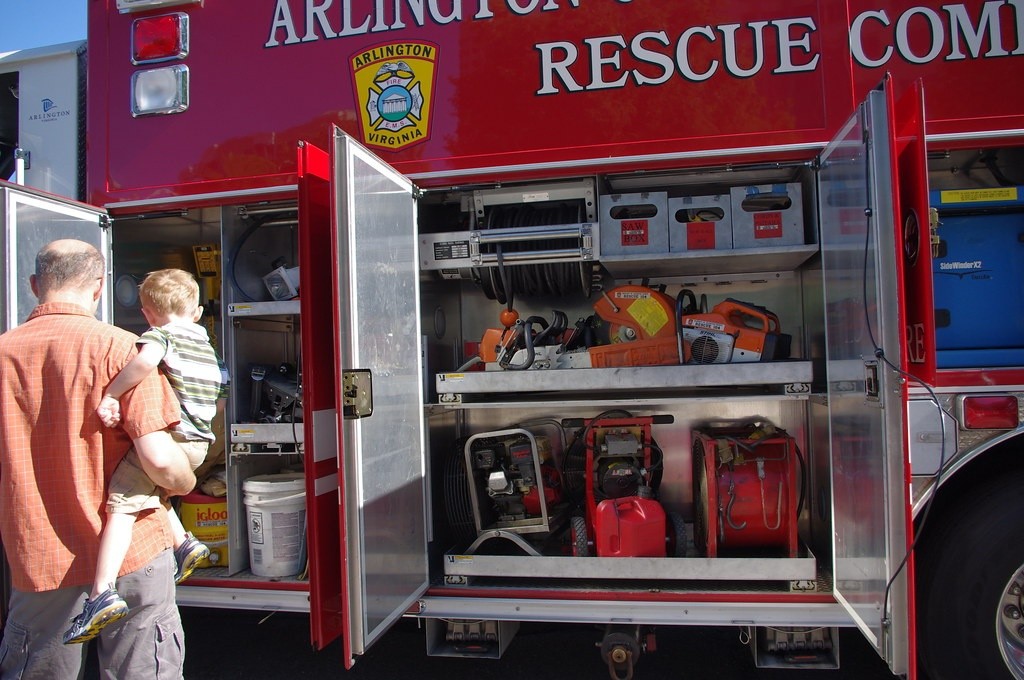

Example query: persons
[0,239,197,680]
[61,267,230,646]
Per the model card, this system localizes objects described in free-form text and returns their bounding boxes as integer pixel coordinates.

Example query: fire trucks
[0,0,1023,680]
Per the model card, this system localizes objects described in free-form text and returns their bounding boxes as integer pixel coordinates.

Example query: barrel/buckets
[242,473,310,576]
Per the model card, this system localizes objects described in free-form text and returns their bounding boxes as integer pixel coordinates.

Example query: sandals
[174,531,210,585]
[62,582,129,644]
